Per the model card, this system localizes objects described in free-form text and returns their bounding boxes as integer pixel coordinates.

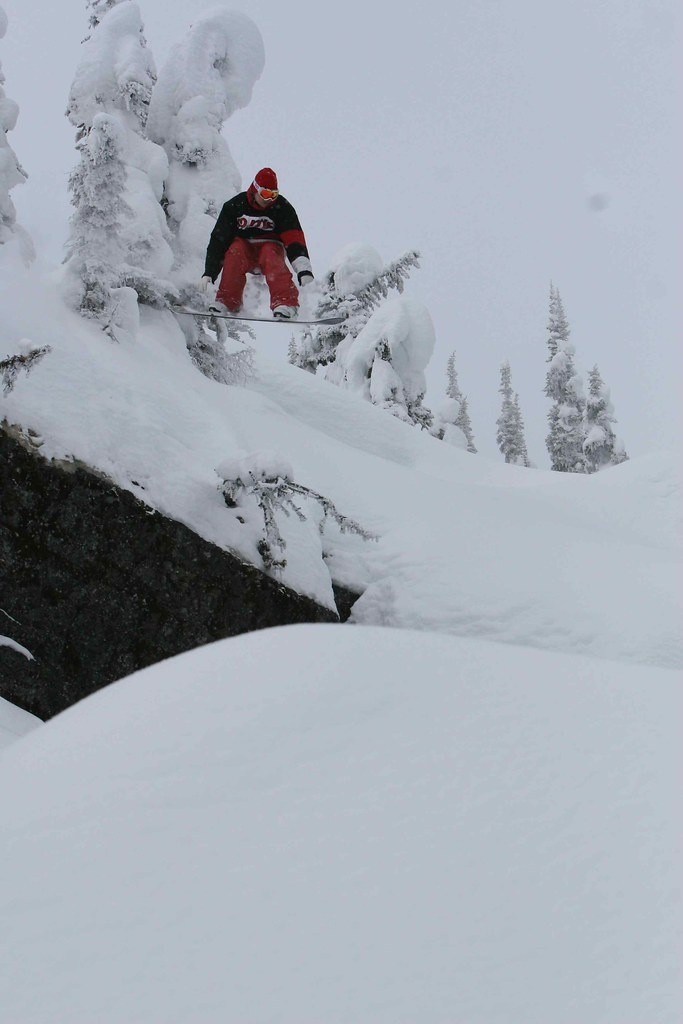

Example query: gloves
[196,276,213,292]
[300,275,313,286]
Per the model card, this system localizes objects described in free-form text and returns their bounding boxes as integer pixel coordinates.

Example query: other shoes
[208,301,228,313]
[272,304,299,318]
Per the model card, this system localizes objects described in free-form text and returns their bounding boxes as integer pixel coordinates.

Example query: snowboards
[169,304,346,325]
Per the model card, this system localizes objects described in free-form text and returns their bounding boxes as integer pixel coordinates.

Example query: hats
[246,167,278,210]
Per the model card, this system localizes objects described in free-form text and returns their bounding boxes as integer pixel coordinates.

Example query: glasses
[252,181,280,201]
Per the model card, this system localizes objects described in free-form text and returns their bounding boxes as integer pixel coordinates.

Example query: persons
[198,168,315,318]
